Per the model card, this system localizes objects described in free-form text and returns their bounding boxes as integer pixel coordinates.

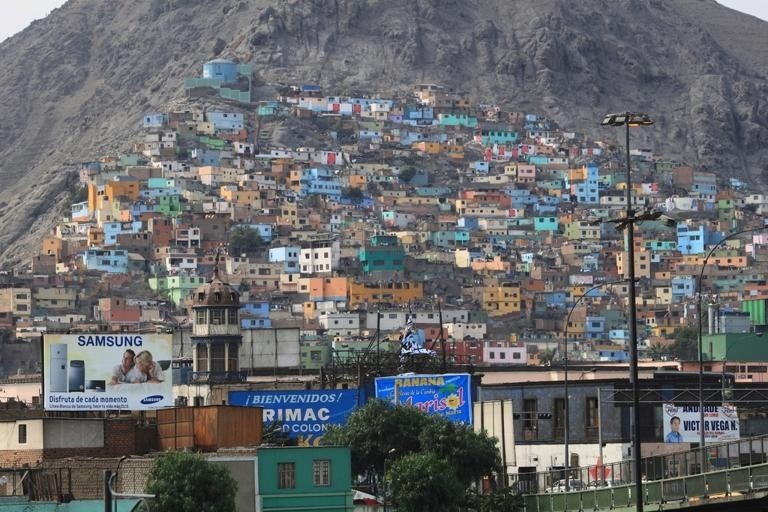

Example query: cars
[545,478,587,493]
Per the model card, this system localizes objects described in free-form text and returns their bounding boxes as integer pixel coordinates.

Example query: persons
[665,416,683,444]
[109,349,164,385]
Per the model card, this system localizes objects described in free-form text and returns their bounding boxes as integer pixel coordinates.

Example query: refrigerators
[49,343,85,393]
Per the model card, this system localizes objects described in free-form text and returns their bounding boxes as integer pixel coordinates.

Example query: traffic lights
[538,413,552,419]
[513,413,520,419]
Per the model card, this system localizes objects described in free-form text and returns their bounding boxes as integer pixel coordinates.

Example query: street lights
[698,222,768,473]
[565,110,654,512]
[115,455,126,512]
